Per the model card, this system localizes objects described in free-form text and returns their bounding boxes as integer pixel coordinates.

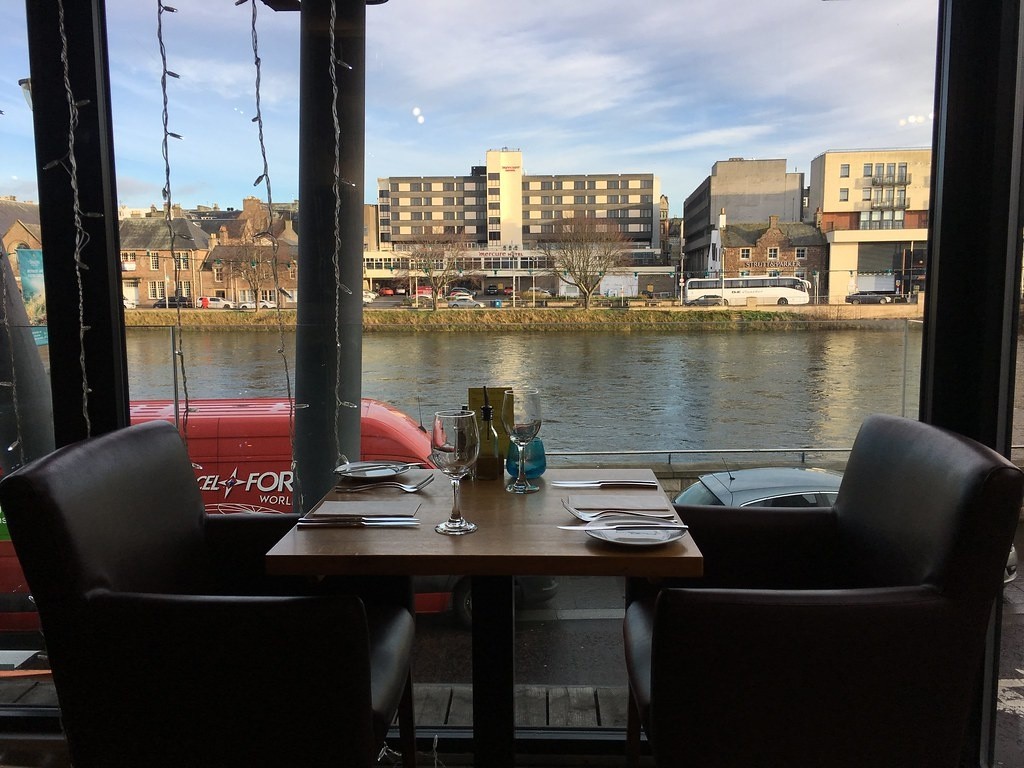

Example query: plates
[585,521,688,546]
[336,461,410,478]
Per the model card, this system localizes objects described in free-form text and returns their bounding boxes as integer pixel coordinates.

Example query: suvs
[487,284,499,295]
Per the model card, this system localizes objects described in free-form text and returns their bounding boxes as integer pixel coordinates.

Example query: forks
[563,502,678,523]
[561,499,674,518]
[335,477,434,492]
[335,474,433,488]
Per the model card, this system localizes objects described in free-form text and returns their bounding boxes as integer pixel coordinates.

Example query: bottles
[454,406,475,479]
[475,406,498,481]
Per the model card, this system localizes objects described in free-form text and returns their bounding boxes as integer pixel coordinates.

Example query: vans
[1,397,450,635]
[153,296,189,308]
[198,297,237,309]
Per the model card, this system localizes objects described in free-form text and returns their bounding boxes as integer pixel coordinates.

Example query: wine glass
[431,409,478,535]
[502,390,542,494]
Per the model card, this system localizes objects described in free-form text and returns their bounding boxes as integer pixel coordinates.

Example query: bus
[685,276,812,305]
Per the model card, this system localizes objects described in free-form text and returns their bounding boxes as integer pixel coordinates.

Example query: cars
[502,287,513,295]
[448,287,478,301]
[240,300,276,309]
[448,297,486,308]
[590,291,606,298]
[508,296,524,304]
[410,294,433,300]
[846,291,890,304]
[392,285,406,294]
[122,295,136,309]
[686,295,723,306]
[377,287,393,297]
[363,288,379,307]
[670,465,1022,584]
[528,287,551,296]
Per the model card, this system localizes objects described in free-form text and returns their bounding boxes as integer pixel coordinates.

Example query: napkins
[311,498,423,518]
[567,494,670,510]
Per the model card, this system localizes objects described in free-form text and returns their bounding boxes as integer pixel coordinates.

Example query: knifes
[334,460,427,472]
[553,480,657,483]
[557,525,688,531]
[550,483,658,490]
[298,517,420,521]
[297,522,422,529]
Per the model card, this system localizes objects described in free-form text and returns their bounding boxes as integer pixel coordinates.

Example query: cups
[507,437,546,478]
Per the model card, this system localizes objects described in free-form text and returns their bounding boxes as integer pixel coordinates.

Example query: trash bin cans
[495,300,501,308]
[542,300,546,308]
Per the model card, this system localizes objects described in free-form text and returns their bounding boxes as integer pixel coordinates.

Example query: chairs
[615,416,1024,768]
[0,420,416,768]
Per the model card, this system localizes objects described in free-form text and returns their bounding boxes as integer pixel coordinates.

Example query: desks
[263,465,706,768]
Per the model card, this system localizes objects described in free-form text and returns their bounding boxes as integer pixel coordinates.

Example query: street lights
[415,259,419,308]
[531,255,535,307]
[721,247,726,306]
[680,253,685,305]
[910,261,923,296]
[511,251,516,307]
